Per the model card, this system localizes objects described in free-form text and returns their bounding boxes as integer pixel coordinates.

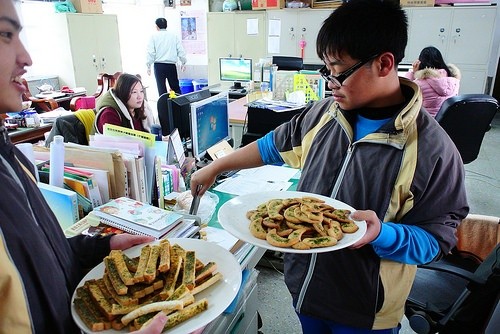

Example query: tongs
[189,183,201,214]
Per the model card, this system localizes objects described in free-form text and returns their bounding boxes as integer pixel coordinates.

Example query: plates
[216,191,367,254]
[70,237,242,334]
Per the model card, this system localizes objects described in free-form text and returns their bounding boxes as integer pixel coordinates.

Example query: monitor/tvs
[166,88,235,161]
[219,57,253,89]
[397,64,413,77]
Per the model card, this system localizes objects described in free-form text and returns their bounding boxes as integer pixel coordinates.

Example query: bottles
[25,111,40,127]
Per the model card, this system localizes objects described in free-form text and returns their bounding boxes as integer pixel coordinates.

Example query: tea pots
[36,84,52,92]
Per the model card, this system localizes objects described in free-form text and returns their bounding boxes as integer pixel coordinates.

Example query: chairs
[156,93,180,135]
[401,242,500,334]
[434,94,498,164]
[56,109,98,146]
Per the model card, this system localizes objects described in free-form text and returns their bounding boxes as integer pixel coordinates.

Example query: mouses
[241,90,246,93]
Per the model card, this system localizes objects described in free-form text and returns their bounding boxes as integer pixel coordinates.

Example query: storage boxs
[70,0,103,13]
[251,0,437,10]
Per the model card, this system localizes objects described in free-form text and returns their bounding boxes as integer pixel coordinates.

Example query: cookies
[76,240,223,331]
[245,196,358,249]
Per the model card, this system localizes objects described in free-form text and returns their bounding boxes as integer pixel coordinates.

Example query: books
[32,123,201,249]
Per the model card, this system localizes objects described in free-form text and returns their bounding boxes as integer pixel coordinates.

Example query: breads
[176,189,215,213]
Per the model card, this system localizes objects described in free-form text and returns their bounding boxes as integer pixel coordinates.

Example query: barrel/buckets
[178,79,208,95]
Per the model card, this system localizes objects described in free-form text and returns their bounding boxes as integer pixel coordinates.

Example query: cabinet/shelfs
[266,8,333,65]
[213,271,259,334]
[410,5,498,94]
[208,12,265,87]
[49,14,119,95]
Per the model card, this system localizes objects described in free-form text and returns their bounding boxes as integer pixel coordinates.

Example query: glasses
[318,53,378,87]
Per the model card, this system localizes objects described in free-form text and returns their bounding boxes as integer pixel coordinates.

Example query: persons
[0,0,206,334]
[405,47,461,118]
[90,73,155,135]
[147,17,187,97]
[190,0,469,334]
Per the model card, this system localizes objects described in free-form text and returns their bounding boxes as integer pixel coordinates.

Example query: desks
[8,106,97,144]
[136,161,303,334]
